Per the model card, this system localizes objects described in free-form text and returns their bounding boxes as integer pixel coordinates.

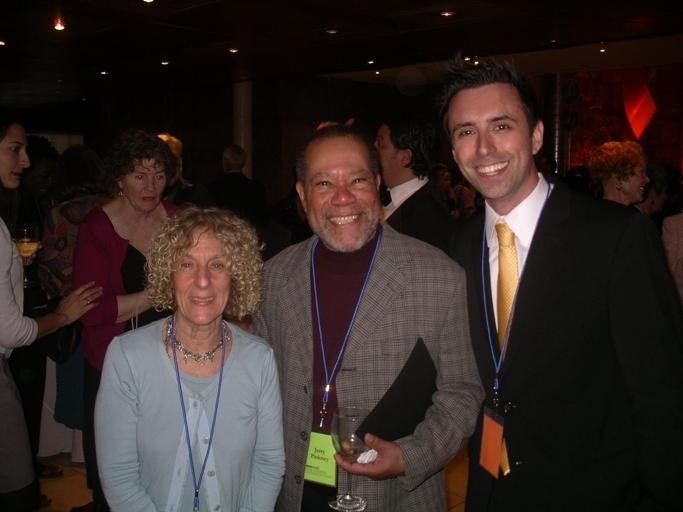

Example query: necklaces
[165,320,232,365]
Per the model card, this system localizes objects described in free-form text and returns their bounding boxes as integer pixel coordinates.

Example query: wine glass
[15,228,40,287]
[328,407,370,511]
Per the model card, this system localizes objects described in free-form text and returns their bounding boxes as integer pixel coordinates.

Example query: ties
[494,225,519,351]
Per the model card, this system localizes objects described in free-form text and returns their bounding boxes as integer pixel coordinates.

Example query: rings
[84,298,90,306]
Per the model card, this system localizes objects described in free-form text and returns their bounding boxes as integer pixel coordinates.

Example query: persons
[630,160,673,217]
[191,142,273,229]
[245,126,488,507]
[433,48,683,506]
[1,108,104,507]
[71,135,186,507]
[368,111,462,255]
[93,203,287,506]
[154,134,184,201]
[3,133,86,478]
[584,136,653,210]
